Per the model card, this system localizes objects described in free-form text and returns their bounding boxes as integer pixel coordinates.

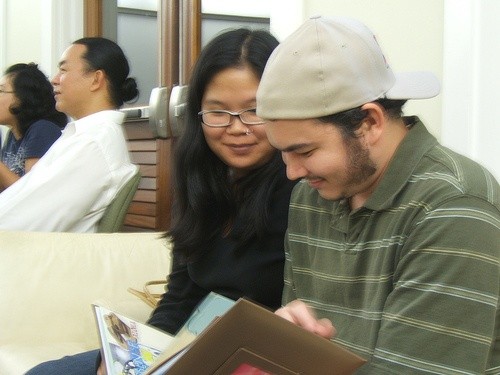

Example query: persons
[256,14,500,375]
[0,37,139,233]
[0,63,61,192]
[24,27,302,375]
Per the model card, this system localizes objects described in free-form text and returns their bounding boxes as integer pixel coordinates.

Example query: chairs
[98,172,140,233]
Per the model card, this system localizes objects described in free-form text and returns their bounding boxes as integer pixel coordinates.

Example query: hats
[257,15,440,119]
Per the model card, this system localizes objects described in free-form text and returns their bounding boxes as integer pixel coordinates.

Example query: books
[92,292,369,375]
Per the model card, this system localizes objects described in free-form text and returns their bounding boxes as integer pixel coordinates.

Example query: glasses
[198,108,266,127]
